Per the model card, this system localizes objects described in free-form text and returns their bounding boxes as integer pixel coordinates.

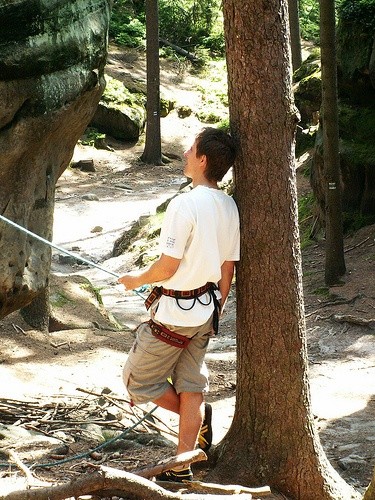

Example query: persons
[116,125,242,485]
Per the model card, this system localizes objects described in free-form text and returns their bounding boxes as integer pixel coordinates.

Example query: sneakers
[155,466,193,482]
[199,403,213,449]
